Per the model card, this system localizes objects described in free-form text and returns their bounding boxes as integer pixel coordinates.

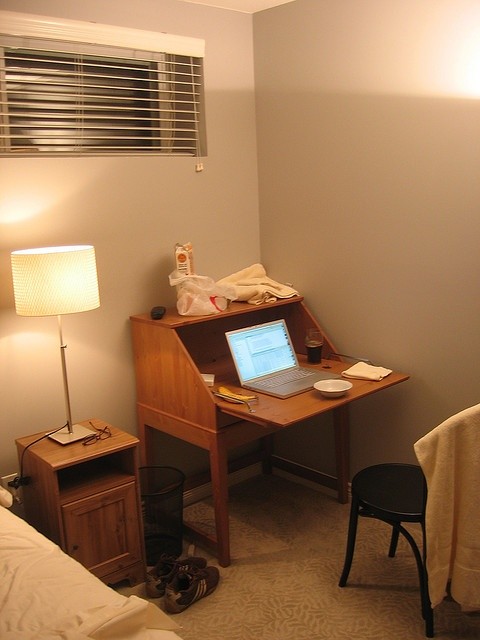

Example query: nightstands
[16,417,149,588]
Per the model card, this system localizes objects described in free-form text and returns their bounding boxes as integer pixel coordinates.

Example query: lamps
[10,244,101,446]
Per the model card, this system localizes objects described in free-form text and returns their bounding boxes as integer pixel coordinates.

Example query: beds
[0,487,183,640]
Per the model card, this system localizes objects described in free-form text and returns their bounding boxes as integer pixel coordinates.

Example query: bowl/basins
[313,378,353,398]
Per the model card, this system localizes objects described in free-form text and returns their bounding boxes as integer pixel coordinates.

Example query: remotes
[151,306,165,319]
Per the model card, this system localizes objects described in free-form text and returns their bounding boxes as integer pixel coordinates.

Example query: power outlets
[0,473,19,501]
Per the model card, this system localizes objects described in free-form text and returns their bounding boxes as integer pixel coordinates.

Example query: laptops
[225,319,341,399]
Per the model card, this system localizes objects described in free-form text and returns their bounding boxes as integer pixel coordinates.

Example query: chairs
[338,463,431,622]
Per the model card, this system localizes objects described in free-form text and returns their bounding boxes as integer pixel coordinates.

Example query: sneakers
[164,565,219,614]
[145,552,207,599]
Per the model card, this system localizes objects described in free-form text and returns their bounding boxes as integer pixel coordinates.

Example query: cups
[304,328,324,365]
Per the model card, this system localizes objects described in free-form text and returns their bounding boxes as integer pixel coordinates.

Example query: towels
[413,402,480,611]
[341,361,392,381]
[216,263,299,305]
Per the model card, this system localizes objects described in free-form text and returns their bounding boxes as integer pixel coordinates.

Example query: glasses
[81,421,111,446]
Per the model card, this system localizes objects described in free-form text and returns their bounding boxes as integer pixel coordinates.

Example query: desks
[130,295,411,568]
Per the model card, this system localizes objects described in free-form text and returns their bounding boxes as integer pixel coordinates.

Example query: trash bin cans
[137,465,184,567]
